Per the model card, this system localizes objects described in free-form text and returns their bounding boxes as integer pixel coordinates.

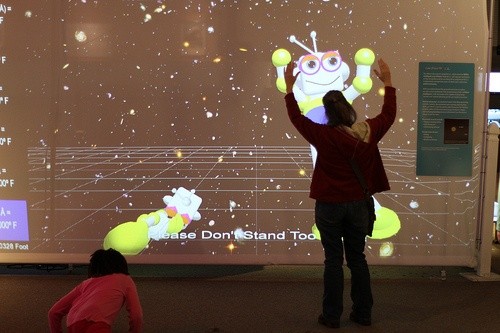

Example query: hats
[321,90,341,108]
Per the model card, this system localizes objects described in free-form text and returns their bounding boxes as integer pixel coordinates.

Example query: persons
[283,59,396,328]
[48,247,143,333]
